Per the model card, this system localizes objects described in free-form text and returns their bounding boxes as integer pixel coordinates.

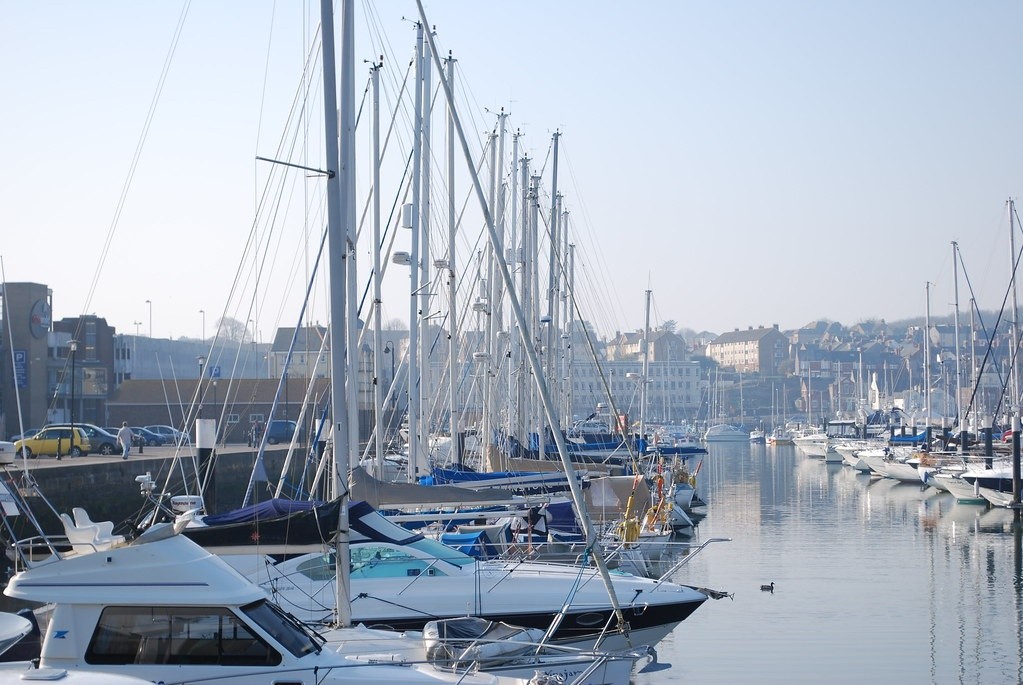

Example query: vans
[263,420,307,445]
[41,423,130,455]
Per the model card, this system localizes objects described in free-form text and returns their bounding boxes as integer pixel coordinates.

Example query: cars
[0,441,16,463]
[10,429,43,443]
[140,424,191,447]
[14,427,92,459]
[130,427,167,446]
[102,427,146,446]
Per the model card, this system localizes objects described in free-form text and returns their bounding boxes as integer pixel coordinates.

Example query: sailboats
[0,0,736,685]
[784,194,1023,508]
[702,366,750,442]
[766,379,790,445]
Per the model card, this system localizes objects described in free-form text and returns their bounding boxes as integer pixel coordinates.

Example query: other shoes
[123,457,127,460]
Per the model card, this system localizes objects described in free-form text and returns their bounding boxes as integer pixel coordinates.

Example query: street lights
[132,322,142,334]
[211,380,221,424]
[195,354,210,417]
[146,300,153,339]
[199,310,206,344]
[65,338,81,458]
[248,319,259,378]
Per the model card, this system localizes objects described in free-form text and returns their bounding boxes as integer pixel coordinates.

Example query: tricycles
[247,428,263,449]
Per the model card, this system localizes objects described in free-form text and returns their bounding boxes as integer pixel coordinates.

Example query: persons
[117,421,134,460]
[251,419,262,449]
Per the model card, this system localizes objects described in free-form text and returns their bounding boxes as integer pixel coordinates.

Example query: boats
[748,419,766,442]
